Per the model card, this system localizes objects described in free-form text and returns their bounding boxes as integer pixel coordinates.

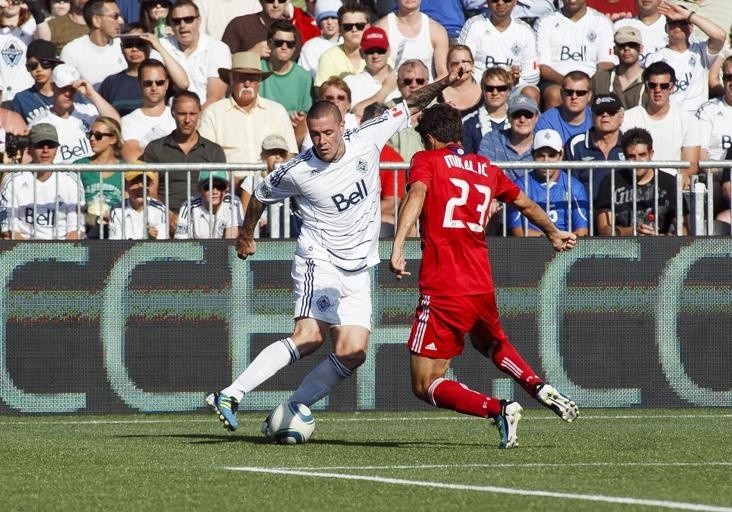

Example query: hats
[50,64,80,87]
[614,26,642,46]
[314,0,343,25]
[196,169,229,181]
[507,94,540,114]
[594,94,624,112]
[218,51,274,83]
[360,27,389,50]
[124,160,153,180]
[261,134,288,151]
[28,123,60,146]
[25,38,63,62]
[533,128,562,153]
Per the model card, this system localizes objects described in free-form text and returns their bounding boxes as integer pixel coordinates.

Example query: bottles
[154,15,167,38]
[646,215,656,227]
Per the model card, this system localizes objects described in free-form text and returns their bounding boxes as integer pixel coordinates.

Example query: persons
[388,102,581,449]
[0,0,732,238]
[204,60,473,437]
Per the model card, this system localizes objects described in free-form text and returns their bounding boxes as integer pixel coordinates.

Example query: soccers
[268,401,316,444]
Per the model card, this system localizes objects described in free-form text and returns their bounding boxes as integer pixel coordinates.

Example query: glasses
[85,131,115,140]
[171,16,199,25]
[561,87,589,97]
[403,76,426,87]
[483,83,509,92]
[644,79,671,89]
[142,79,165,88]
[26,60,53,71]
[274,39,297,48]
[342,21,365,31]
[326,96,346,101]
[203,182,227,191]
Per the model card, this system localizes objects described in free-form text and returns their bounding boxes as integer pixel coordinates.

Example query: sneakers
[494,400,524,449]
[205,392,240,430]
[533,382,580,423]
